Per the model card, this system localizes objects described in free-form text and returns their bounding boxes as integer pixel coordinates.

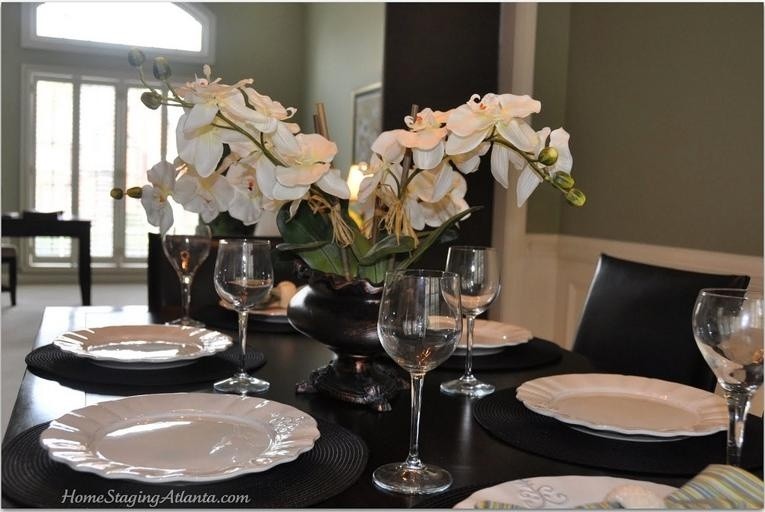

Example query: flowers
[109,46,585,290]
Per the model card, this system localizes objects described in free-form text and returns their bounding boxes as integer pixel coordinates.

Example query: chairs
[570,254,750,395]
[141,229,291,306]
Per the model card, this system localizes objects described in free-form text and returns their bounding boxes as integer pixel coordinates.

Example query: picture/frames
[349,81,384,167]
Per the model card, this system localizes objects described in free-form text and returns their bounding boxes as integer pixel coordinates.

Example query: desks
[1,209,93,306]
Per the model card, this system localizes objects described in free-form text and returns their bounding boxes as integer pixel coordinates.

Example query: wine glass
[691,287,765,467]
[213,239,274,394]
[439,244,501,399]
[162,223,211,328]
[372,269,463,495]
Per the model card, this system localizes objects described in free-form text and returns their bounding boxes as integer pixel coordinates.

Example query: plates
[218,299,290,323]
[53,324,234,371]
[39,390,322,487]
[423,315,533,356]
[452,473,681,509]
[515,371,730,442]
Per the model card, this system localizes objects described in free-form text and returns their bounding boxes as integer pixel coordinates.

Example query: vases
[287,270,432,409]
